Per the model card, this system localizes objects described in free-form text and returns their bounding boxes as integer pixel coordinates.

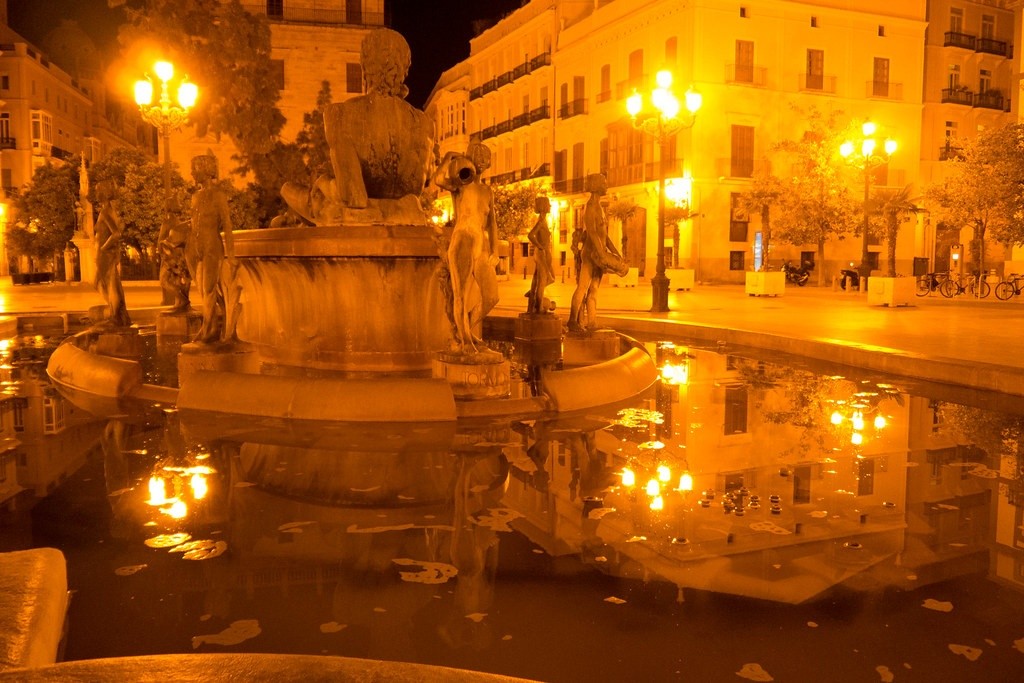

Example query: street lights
[131,57,200,305]
[625,68,705,314]
[839,122,899,292]
[620,339,692,512]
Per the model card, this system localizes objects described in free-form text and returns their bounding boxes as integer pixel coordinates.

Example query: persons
[94,180,133,328]
[279,27,440,226]
[448,142,503,361]
[523,197,556,315]
[568,174,632,335]
[156,155,233,341]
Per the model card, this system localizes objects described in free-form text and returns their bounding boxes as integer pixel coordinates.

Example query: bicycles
[916,270,991,299]
[994,272,1024,301]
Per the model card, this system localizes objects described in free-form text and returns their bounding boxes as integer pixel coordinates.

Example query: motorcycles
[779,257,815,287]
[840,264,875,290]
